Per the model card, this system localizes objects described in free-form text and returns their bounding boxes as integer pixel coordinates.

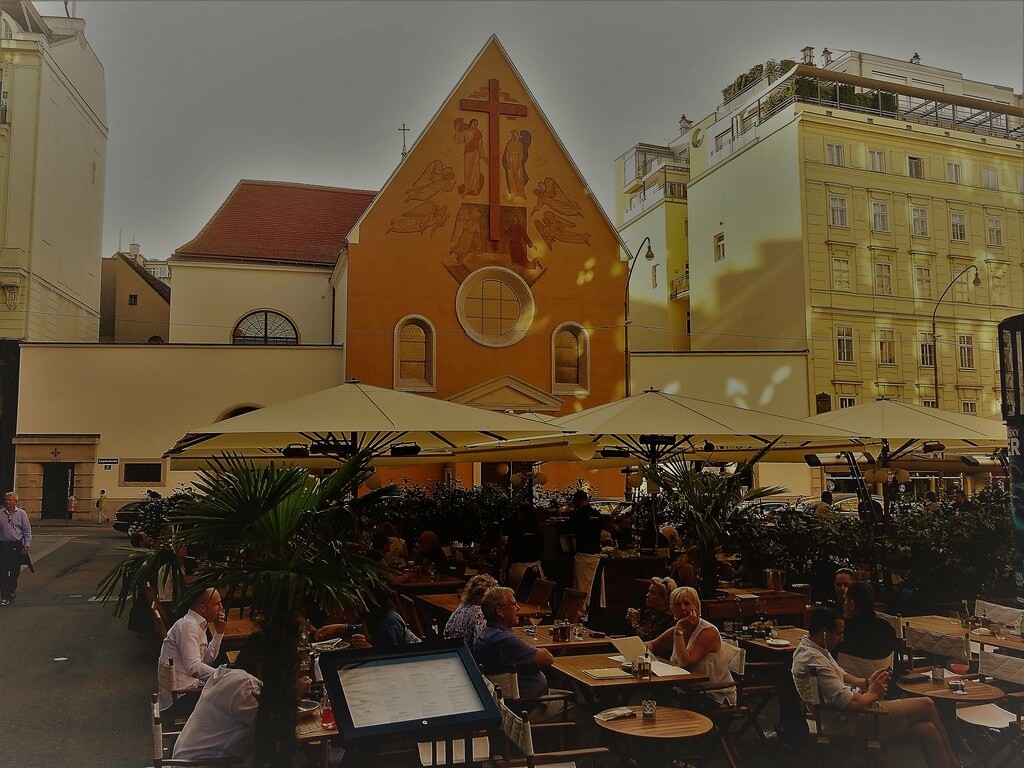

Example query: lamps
[627,466,675,488]
[363,471,382,490]
[496,463,547,484]
[887,439,946,459]
[862,466,910,484]
[600,434,715,461]
[282,438,422,456]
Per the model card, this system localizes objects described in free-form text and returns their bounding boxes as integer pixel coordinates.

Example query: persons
[130,490,979,768]
[0,492,32,606]
[95,490,109,524]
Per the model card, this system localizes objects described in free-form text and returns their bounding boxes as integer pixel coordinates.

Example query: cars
[112,501,155,536]
[556,494,923,525]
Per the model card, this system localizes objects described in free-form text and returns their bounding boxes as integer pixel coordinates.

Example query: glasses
[661,580,670,593]
[501,601,519,610]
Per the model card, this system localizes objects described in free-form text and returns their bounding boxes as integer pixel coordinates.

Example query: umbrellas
[163,376,1008,512]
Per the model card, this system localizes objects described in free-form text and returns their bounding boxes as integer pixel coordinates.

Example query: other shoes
[328,746,346,767]
[106,518,109,524]
[577,732,610,749]
[756,728,779,744]
[0,597,10,606]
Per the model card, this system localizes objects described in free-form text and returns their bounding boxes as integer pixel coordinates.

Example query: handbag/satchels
[96,500,99,508]
[406,629,422,643]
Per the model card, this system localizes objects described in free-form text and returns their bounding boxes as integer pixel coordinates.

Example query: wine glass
[530,618,542,640]
[950,664,970,695]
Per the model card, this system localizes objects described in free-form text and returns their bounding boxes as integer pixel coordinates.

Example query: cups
[632,660,642,674]
[428,570,436,582]
[723,621,732,634]
[948,611,959,624]
[642,700,656,720]
[932,668,944,685]
[573,623,585,641]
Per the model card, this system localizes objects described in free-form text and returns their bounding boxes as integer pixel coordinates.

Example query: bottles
[754,622,766,641]
[960,601,970,628]
[614,539,618,556]
[995,621,1007,639]
[1021,616,1024,642]
[561,619,570,641]
[553,618,560,641]
[772,619,779,639]
[640,652,651,678]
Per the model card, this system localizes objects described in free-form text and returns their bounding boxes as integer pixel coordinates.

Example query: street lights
[624,236,655,398]
[931,265,982,410]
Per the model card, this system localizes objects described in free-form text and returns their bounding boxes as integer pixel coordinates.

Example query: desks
[395,572,466,599]
[594,706,714,768]
[719,627,809,653]
[207,603,318,651]
[899,612,1024,685]
[508,622,616,657]
[895,676,1006,762]
[715,588,785,596]
[549,651,713,717]
[415,593,552,637]
[289,692,339,768]
[180,575,253,600]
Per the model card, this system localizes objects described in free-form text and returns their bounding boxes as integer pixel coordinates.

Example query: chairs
[145,521,1024,768]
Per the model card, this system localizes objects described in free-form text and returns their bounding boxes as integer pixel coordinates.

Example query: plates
[767,639,790,646]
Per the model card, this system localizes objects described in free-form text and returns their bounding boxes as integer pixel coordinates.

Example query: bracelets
[674,629,683,635]
[865,677,869,688]
[632,623,640,629]
